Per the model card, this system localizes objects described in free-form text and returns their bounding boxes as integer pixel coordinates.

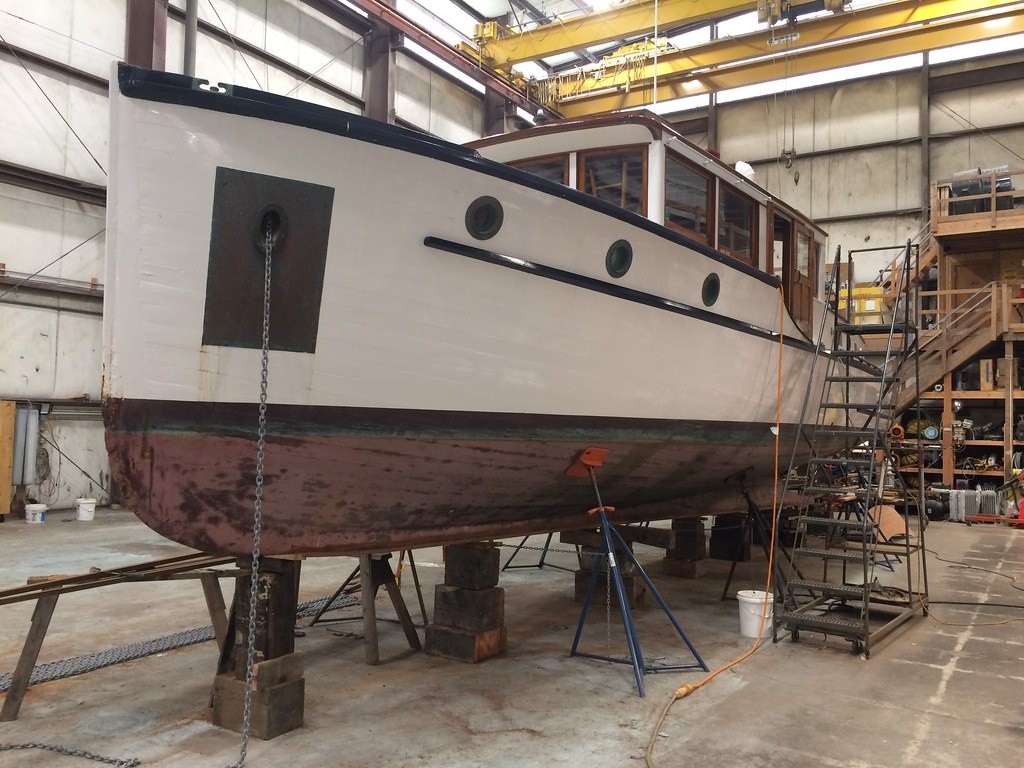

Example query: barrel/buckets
[77,498,97,520]
[25,503,47,523]
[737,589,774,640]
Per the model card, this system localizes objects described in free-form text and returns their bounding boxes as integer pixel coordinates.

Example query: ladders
[771,238,931,659]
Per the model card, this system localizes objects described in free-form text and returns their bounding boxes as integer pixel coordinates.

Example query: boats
[99,57,895,564]
